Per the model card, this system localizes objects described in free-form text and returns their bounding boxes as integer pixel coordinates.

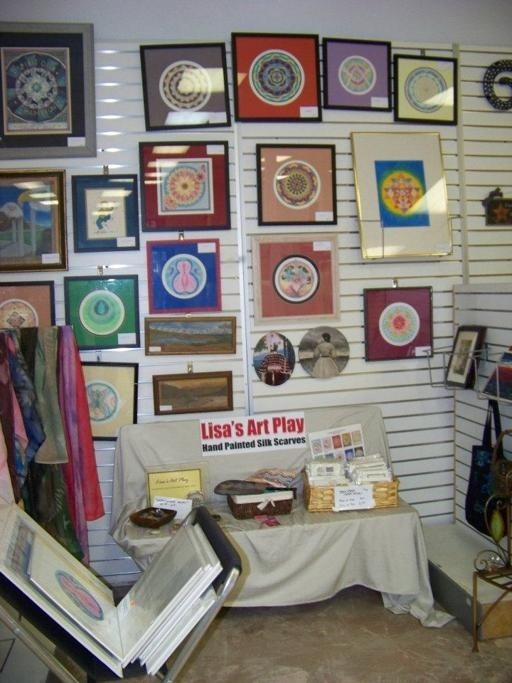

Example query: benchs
[111,406,457,628]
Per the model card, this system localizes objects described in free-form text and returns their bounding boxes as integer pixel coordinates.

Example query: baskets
[226,494,293,520]
[301,468,400,513]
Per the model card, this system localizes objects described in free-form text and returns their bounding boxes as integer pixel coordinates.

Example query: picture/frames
[76,361,140,443]
[147,468,202,508]
[146,239,223,315]
[364,285,435,362]
[0,281,55,332]
[251,233,341,326]
[231,31,323,125]
[144,317,238,356]
[321,38,393,114]
[0,168,70,273]
[349,130,455,259]
[65,274,141,350]
[443,326,487,390]
[71,173,140,253]
[152,371,234,416]
[140,42,232,131]
[139,140,232,233]
[0,19,98,161]
[393,53,459,127]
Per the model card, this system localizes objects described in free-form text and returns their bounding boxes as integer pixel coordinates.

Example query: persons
[314,333,342,377]
[95,200,115,231]
[259,336,290,386]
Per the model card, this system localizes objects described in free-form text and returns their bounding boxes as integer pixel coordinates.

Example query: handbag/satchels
[465,399,509,538]
